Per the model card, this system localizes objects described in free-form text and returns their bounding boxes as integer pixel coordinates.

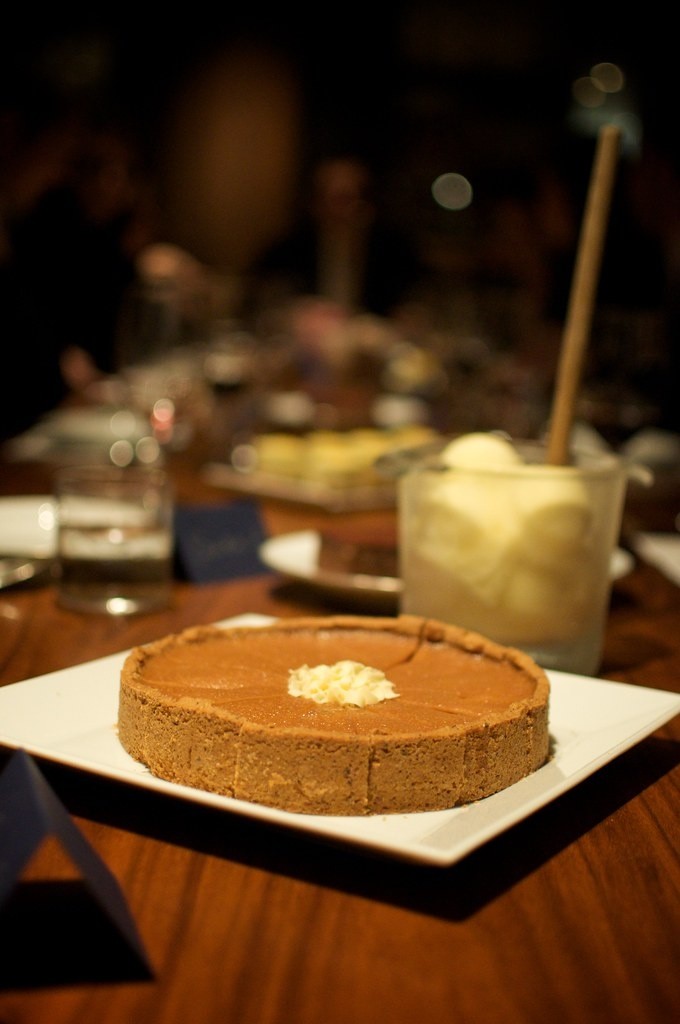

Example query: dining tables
[0,316,680,1024]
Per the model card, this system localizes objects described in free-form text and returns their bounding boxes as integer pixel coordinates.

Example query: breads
[313,525,397,579]
[116,614,551,814]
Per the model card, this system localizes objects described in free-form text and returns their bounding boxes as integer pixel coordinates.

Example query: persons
[0,96,679,394]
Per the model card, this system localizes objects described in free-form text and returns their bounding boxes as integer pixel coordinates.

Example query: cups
[394,441,626,676]
[49,428,175,616]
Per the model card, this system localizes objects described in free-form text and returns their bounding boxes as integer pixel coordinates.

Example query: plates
[259,529,402,602]
[0,610,679,867]
[0,494,172,560]
